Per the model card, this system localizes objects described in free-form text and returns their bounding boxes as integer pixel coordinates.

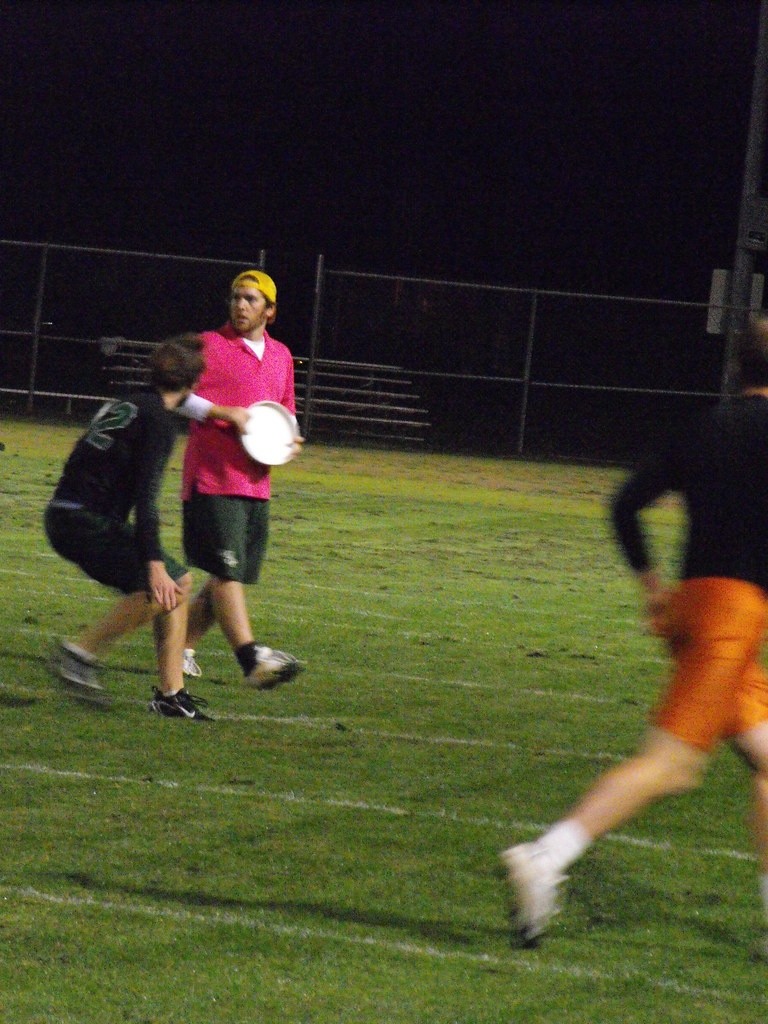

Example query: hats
[230,270,277,304]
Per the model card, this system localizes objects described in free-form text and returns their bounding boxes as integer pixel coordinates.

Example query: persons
[41,338,216,722]
[496,315,768,968]
[174,269,308,690]
[415,260,476,327]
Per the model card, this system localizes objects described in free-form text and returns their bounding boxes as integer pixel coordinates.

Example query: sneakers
[494,843,571,949]
[149,686,217,721]
[46,641,113,710]
[183,648,203,679]
[247,644,308,689]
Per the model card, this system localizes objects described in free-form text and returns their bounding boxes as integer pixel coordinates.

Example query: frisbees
[238,401,301,466]
